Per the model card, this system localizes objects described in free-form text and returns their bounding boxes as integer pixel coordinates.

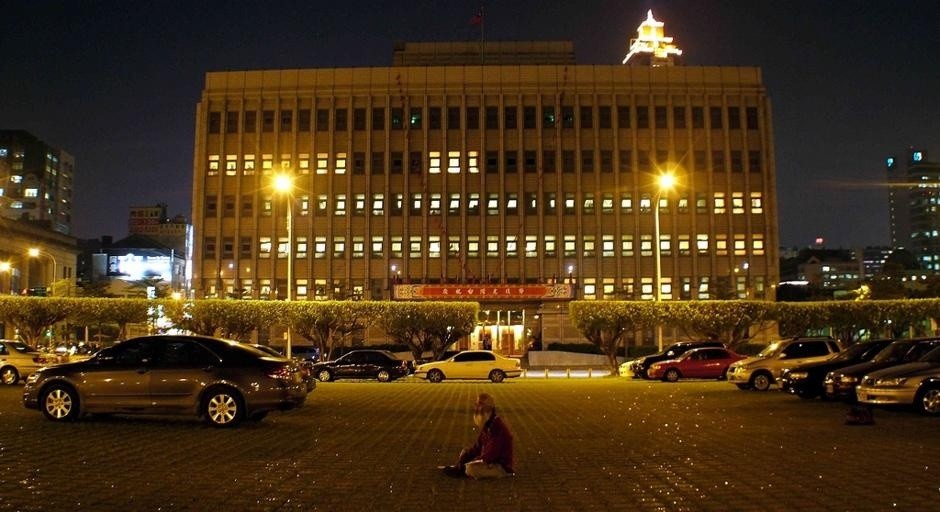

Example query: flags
[464,7,483,25]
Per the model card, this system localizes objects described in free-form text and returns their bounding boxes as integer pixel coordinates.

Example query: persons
[482,333,493,350]
[443,391,516,481]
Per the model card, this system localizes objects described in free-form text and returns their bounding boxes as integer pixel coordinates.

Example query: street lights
[29,248,57,296]
[275,172,295,300]
[653,168,680,352]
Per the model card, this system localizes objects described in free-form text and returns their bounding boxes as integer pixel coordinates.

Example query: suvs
[727,335,845,395]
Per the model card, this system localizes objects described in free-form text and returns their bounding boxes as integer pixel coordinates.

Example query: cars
[647,347,748,382]
[0,338,61,386]
[781,338,940,417]
[22,335,308,428]
[630,341,726,381]
[413,349,523,382]
[311,350,410,383]
